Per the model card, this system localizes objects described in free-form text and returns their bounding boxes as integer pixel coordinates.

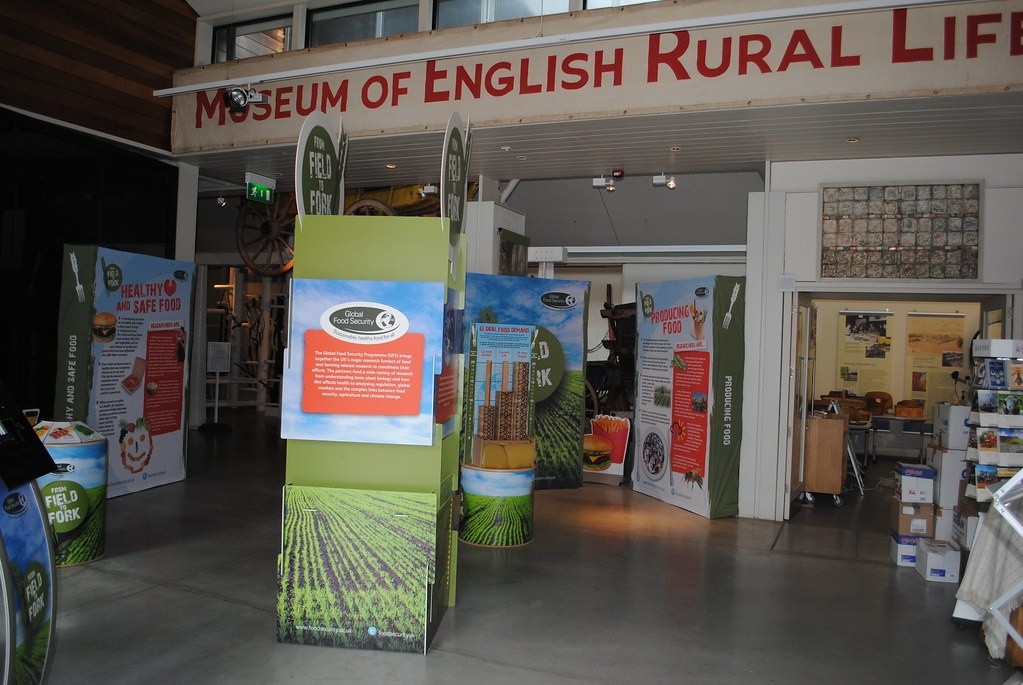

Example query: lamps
[419,182,439,198]
[591,174,617,191]
[217,196,228,207]
[653,173,677,189]
[224,88,267,110]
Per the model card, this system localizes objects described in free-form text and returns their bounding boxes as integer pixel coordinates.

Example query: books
[965,359,1023,503]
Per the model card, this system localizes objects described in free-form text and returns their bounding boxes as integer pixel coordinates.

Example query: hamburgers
[582,435,613,471]
[93,312,117,343]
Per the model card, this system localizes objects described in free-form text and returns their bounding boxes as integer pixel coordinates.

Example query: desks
[869,414,928,465]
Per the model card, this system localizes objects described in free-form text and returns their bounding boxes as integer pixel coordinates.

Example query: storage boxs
[888,338,1023,583]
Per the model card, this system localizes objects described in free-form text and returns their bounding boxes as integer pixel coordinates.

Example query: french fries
[591,415,629,434]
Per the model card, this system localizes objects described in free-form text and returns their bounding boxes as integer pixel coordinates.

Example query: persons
[177,325,183,362]
[246,298,261,323]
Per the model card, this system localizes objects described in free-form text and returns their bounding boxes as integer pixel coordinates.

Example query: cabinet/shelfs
[803,412,849,494]
